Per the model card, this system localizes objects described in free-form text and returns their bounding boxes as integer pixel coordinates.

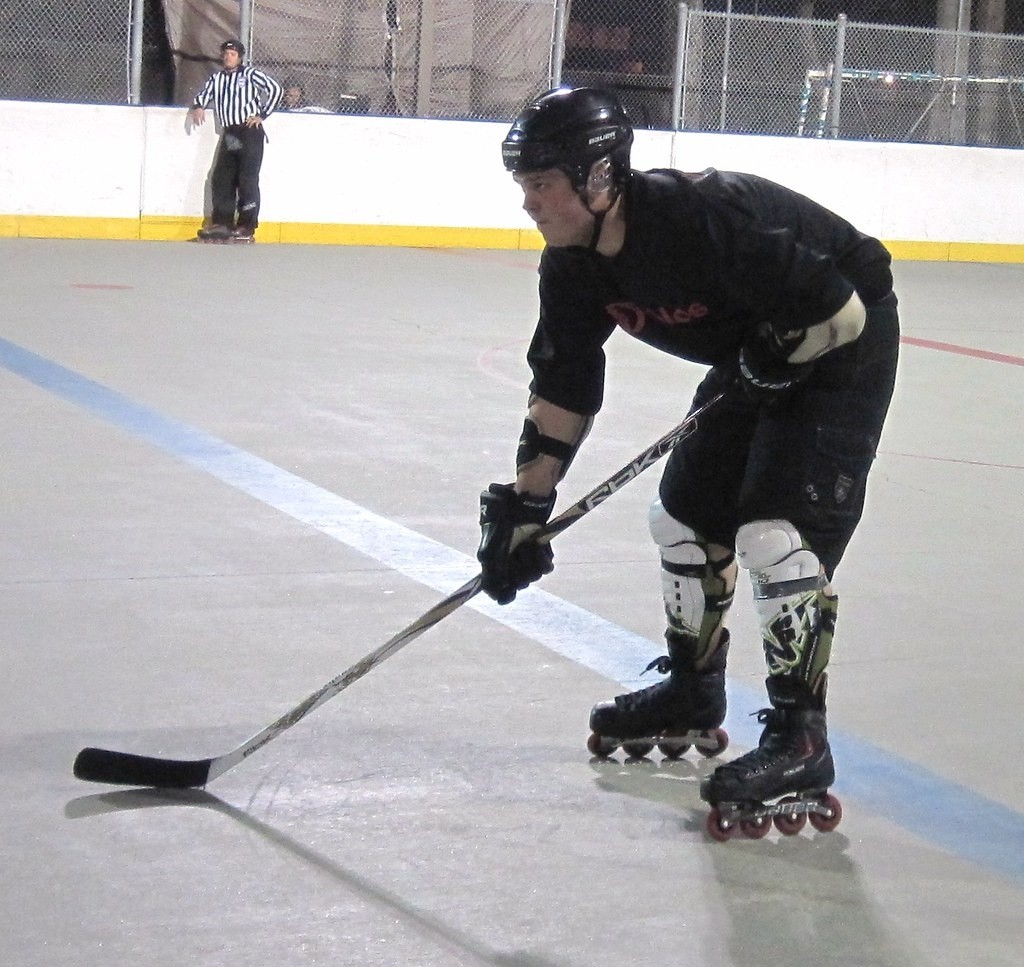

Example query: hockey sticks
[73,385,757,789]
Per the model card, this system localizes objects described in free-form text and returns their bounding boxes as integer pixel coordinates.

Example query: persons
[478,88,900,842]
[275,76,371,114]
[192,41,284,243]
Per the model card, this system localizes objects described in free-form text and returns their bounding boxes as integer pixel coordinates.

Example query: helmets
[220,40,247,59]
[502,85,633,193]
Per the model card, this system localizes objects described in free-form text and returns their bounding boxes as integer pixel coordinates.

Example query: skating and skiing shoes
[588,627,729,758]
[224,225,256,245]
[700,672,842,842]
[197,224,234,244]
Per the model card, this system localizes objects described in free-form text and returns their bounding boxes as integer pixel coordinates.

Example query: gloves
[476,481,554,605]
[736,321,793,407]
